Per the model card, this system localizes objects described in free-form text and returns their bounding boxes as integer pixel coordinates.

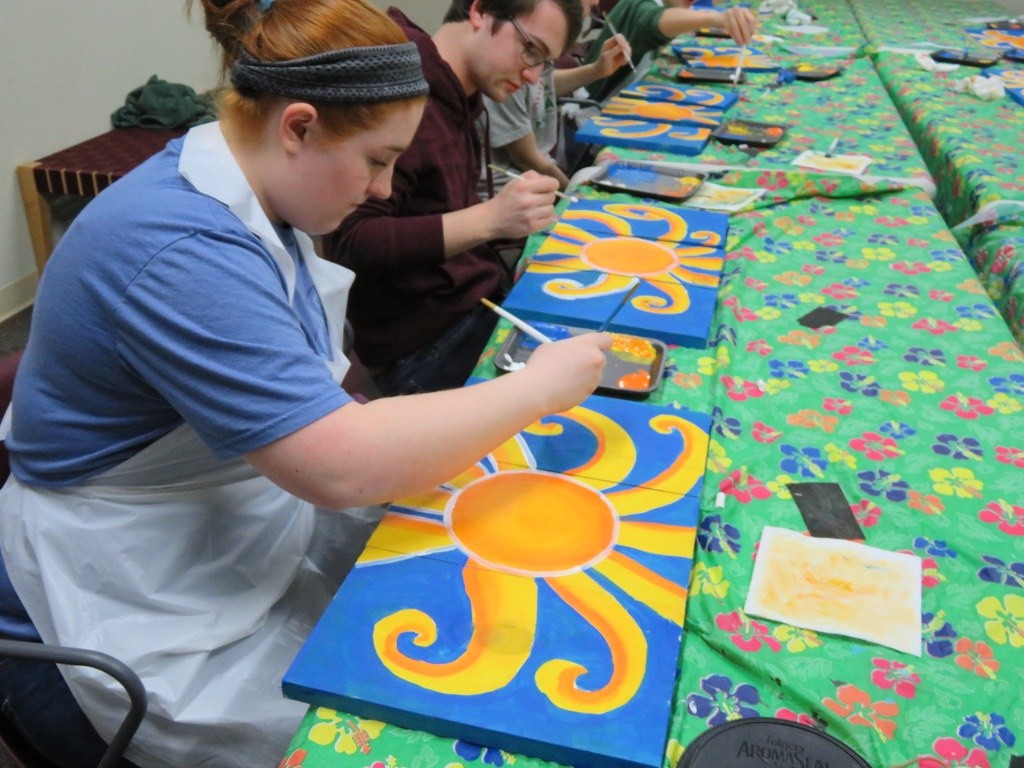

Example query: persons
[582,0,756,100]
[475,0,633,198]
[322,0,584,397]
[0,0,613,768]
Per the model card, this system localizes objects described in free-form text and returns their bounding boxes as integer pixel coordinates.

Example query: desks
[15,91,227,278]
[275,0,1024,768]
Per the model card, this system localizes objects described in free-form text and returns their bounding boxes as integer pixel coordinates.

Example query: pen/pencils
[825,139,840,157]
[734,9,758,87]
[597,281,640,333]
[488,164,578,203]
[481,297,553,344]
[602,11,637,73]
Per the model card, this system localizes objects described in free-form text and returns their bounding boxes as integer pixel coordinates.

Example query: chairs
[0,317,376,768]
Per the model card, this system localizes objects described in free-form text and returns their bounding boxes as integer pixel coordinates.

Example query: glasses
[508,16,558,76]
